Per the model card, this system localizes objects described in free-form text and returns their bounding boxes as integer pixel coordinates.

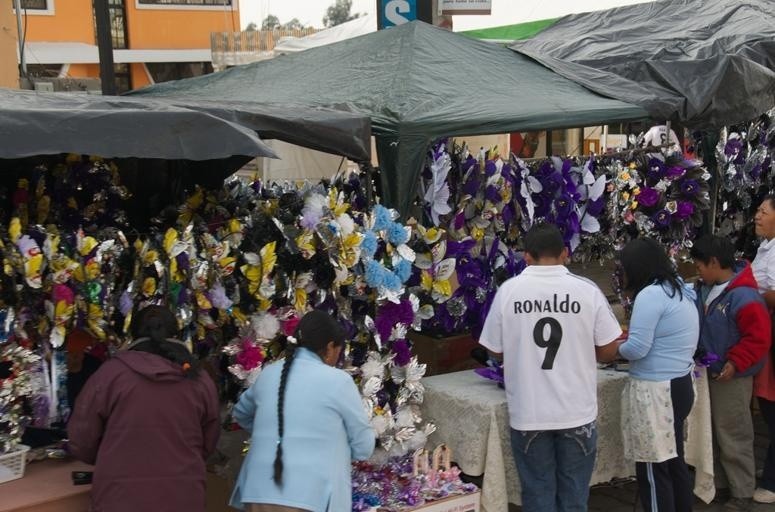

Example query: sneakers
[752,468,775,505]
[721,496,751,512]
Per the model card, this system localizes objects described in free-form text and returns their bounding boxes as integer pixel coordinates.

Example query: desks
[0,398,249,512]
[418,360,717,511]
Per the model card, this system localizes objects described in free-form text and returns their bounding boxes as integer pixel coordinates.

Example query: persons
[749,194,775,504]
[688,233,773,512]
[65,304,221,512]
[227,308,377,512]
[640,119,683,158]
[478,221,624,511]
[617,236,701,512]
